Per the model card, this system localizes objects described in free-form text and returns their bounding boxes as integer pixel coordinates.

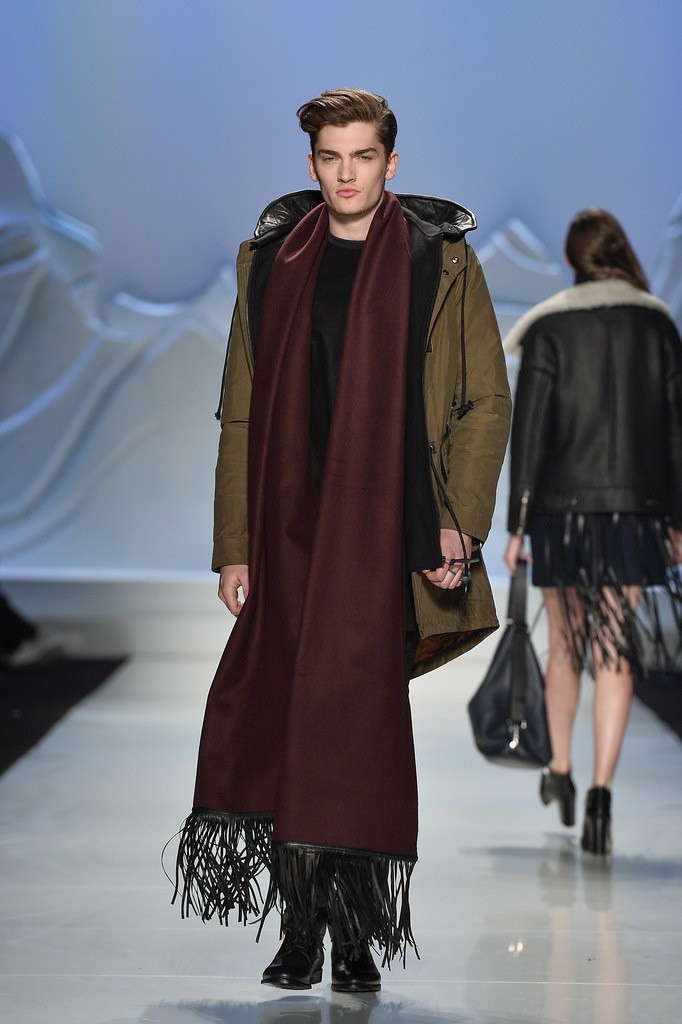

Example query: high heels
[539,767,576,826]
[581,786,613,854]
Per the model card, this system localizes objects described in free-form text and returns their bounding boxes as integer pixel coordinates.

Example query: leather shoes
[260,932,324,990]
[330,935,381,993]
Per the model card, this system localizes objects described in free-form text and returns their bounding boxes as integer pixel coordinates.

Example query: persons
[163,88,513,992]
[499,207,681,858]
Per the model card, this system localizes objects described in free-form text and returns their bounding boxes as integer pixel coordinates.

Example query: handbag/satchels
[465,560,575,770]
